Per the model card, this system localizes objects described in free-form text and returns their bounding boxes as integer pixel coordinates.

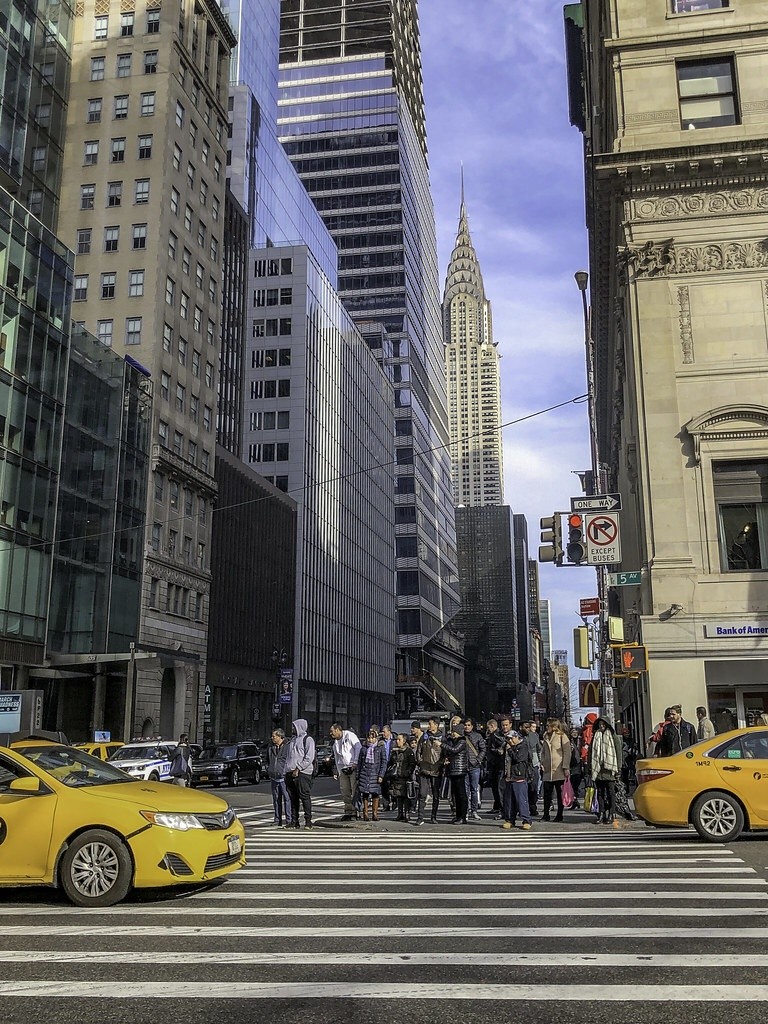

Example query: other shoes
[453,817,467,824]
[373,815,379,821]
[305,823,313,829]
[472,813,481,820]
[271,822,281,826]
[448,817,458,824]
[431,816,439,824]
[594,812,604,824]
[392,800,397,810]
[415,818,424,826]
[351,815,357,820]
[393,815,403,821]
[364,813,370,821]
[381,806,390,812]
[283,822,300,829]
[341,815,351,821]
[487,804,563,830]
[605,814,614,823]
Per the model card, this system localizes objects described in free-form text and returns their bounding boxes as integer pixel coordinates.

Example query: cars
[312,744,335,778]
[632,725,768,842]
[0,735,247,907]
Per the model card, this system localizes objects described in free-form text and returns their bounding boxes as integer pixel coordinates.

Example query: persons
[356,729,387,821]
[755,713,768,759]
[519,713,640,824]
[433,724,468,825]
[652,703,715,757]
[407,737,420,814]
[410,721,426,747]
[379,725,397,811]
[283,719,315,830]
[281,678,291,693]
[386,733,416,822]
[450,715,532,829]
[330,723,362,821]
[168,733,190,787]
[416,716,446,826]
[265,728,291,827]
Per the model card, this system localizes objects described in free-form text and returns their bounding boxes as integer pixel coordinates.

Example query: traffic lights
[620,645,648,672]
[539,515,563,564]
[566,514,585,562]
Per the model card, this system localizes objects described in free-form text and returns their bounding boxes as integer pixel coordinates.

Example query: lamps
[671,603,683,610]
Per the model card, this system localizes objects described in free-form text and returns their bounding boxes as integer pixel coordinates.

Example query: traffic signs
[570,492,623,513]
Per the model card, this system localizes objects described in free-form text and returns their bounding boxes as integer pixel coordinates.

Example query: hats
[585,713,597,724]
[452,724,464,735]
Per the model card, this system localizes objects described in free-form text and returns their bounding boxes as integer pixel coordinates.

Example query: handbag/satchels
[584,780,600,814]
[168,748,184,778]
[341,767,355,775]
[560,775,574,807]
[406,781,416,798]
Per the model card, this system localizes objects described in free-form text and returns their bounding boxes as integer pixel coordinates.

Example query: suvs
[66,738,269,789]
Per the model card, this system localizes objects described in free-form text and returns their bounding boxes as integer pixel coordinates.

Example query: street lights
[270,647,287,731]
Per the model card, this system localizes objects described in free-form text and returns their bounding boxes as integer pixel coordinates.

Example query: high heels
[568,799,580,810]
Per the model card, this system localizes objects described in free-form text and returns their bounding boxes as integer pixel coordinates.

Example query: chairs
[726,546,749,569]
